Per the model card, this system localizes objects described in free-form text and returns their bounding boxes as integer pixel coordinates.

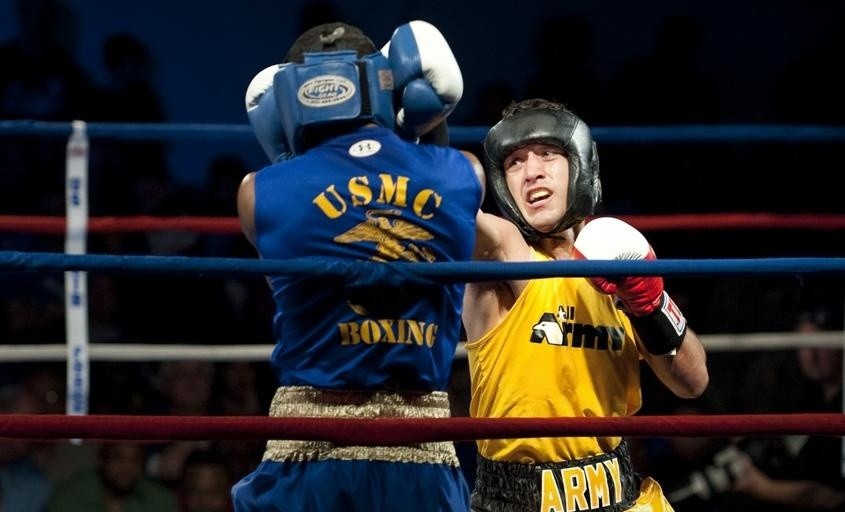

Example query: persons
[227,20,490,512]
[3,31,844,512]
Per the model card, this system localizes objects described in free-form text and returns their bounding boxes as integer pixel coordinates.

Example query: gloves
[245,64,293,163]
[388,20,465,146]
[573,217,688,355]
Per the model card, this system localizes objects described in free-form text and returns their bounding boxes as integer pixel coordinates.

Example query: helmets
[484,98,602,241]
[274,23,395,154]
